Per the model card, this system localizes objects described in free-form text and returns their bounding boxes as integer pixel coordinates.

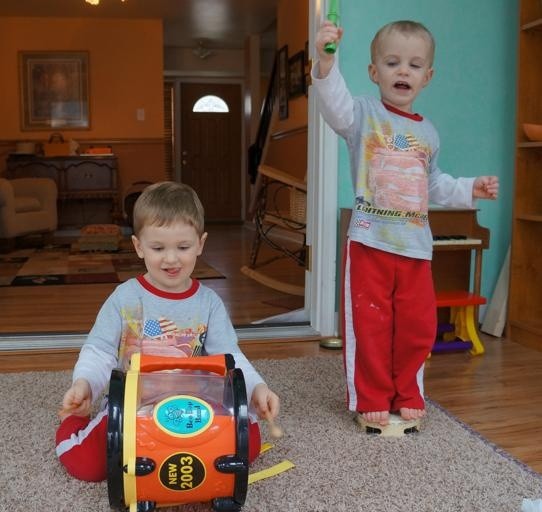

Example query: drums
[107,353,249,507]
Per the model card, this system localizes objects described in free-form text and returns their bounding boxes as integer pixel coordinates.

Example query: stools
[432,290,485,357]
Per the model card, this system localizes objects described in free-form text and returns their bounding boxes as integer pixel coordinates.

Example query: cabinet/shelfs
[505,0,542,354]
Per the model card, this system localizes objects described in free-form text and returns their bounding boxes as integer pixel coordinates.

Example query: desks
[5,154,121,225]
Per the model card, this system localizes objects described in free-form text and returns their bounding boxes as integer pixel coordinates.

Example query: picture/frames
[17,49,92,132]
[276,44,287,120]
[287,50,306,100]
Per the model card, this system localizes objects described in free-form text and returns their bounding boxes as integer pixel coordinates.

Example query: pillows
[81,223,123,235]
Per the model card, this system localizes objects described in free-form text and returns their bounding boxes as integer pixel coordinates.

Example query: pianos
[338,207,490,336]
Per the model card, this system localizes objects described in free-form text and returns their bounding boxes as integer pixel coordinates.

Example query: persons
[310,19,501,427]
[53,182,281,484]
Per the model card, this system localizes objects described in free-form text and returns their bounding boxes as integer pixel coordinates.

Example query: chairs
[241,164,307,296]
[0,177,58,239]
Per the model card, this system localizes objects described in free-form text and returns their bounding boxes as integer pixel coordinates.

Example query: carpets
[1,355,542,511]
[0,236,227,288]
